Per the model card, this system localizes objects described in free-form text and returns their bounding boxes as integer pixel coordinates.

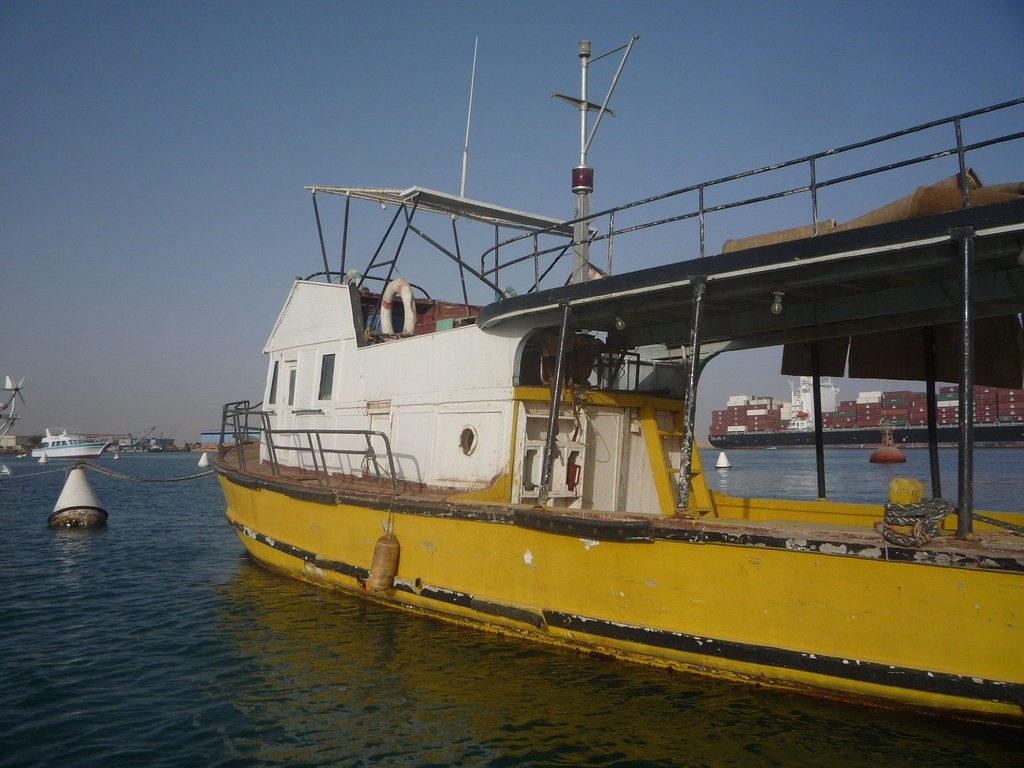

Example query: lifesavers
[378,277,417,340]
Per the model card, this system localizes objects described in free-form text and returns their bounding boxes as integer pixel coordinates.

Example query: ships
[196,36,1023,725]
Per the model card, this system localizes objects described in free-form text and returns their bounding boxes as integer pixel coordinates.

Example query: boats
[31,428,114,458]
